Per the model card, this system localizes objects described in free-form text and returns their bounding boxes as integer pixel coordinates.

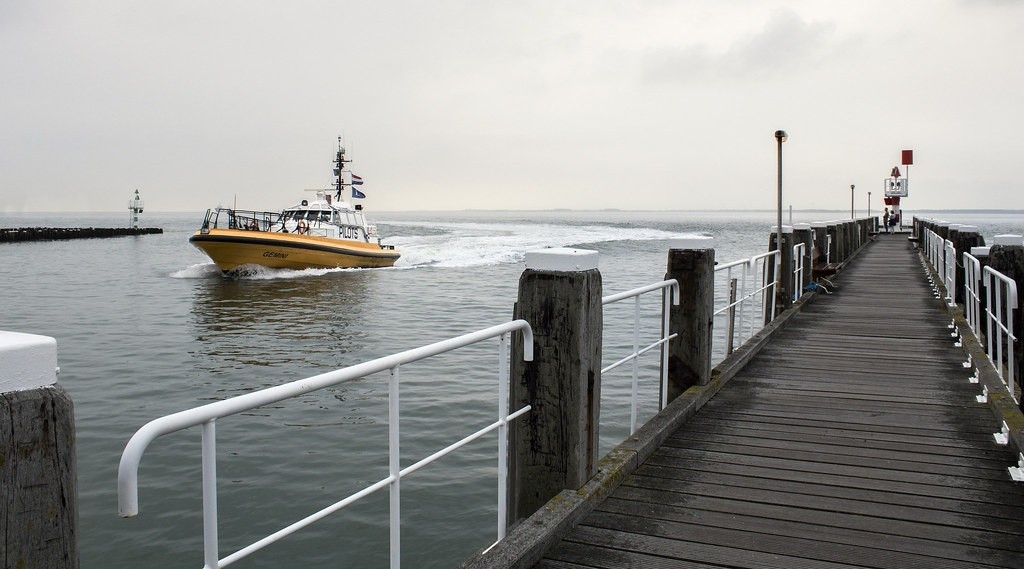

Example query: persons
[883,208,889,234]
[889,210,896,235]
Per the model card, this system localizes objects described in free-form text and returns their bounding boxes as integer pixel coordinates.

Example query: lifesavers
[249,220,257,230]
[297,220,307,235]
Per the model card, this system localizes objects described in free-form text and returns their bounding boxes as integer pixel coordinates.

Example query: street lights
[774,129,789,312]
[867,191,871,218]
[850,184,855,220]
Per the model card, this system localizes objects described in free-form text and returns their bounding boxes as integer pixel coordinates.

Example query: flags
[352,174,365,198]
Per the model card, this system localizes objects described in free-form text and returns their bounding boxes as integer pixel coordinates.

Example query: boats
[188,133,401,278]
